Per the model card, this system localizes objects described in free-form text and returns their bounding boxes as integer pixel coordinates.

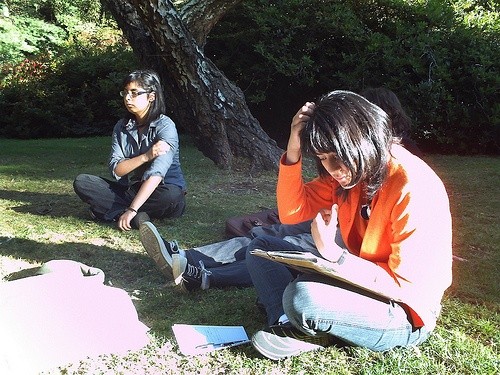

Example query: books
[173,324,252,357]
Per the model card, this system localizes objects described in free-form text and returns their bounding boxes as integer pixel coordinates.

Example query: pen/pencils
[220,340,246,347]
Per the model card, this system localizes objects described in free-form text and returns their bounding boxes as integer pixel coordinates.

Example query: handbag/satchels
[224,209,282,237]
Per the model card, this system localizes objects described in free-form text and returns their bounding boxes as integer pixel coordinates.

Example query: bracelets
[127,207,136,214]
[339,249,348,265]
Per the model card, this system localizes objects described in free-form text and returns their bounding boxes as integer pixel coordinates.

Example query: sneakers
[250,325,330,360]
[138,221,181,282]
[172,253,213,296]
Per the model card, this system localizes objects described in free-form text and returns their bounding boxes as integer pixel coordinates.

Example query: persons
[245,90,452,361]
[141,88,411,295]
[73,70,187,230]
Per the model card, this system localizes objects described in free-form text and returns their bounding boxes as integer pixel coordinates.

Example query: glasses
[119,91,151,97]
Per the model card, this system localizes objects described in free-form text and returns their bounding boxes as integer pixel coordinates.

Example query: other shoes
[132,212,151,229]
[88,206,101,220]
